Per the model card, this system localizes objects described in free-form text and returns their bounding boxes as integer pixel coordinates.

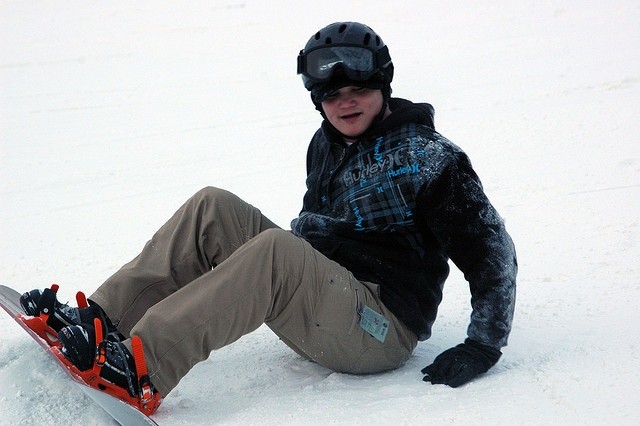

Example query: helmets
[297,21,394,110]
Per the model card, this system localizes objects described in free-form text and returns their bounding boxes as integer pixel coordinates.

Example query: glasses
[297,44,392,82]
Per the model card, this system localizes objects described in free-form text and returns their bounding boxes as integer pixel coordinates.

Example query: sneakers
[57,324,163,404]
[20,289,122,341]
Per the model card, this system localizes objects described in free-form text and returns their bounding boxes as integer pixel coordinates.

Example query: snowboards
[0,285,157,426]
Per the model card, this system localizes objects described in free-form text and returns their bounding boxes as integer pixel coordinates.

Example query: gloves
[421,338,503,389]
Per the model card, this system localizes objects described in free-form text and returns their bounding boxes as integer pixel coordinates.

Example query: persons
[19,20,519,417]
